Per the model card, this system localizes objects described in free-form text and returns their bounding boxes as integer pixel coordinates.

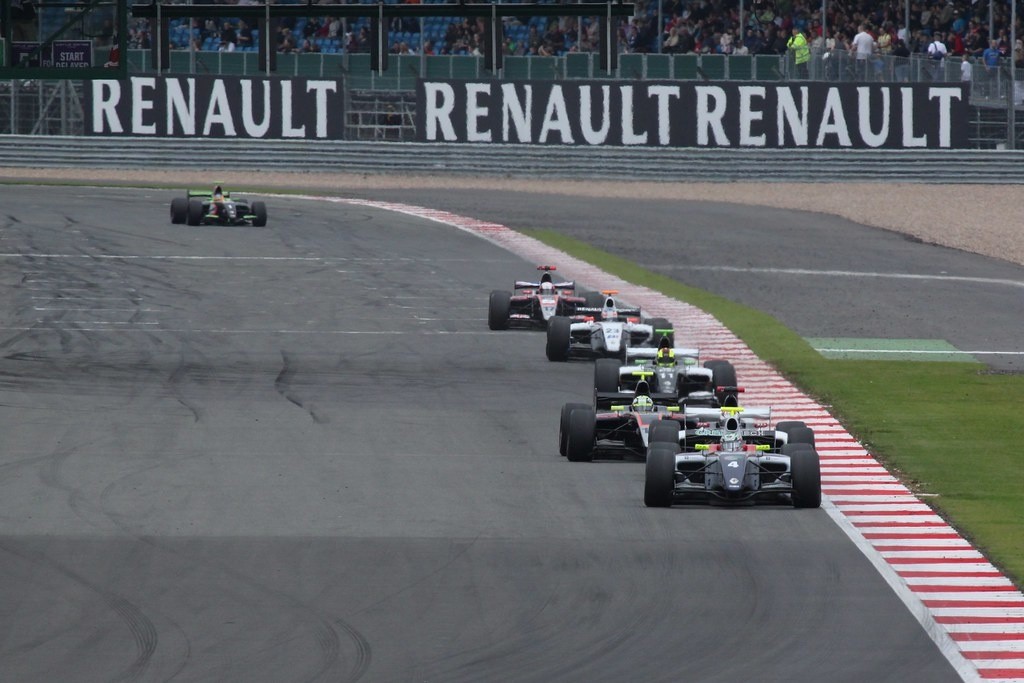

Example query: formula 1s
[589,327,738,410]
[489,262,604,329]
[645,384,822,513]
[559,370,721,463]
[172,183,268,227]
[547,290,676,362]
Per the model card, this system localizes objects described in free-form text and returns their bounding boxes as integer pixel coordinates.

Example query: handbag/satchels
[928,51,943,65]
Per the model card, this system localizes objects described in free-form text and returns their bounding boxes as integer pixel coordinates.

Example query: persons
[720,433,744,453]
[656,347,677,367]
[61,0,1024,100]
[538,282,556,295]
[601,307,618,321]
[631,395,655,414]
[213,193,224,202]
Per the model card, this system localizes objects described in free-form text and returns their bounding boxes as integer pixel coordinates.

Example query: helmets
[656,347,676,367]
[631,394,653,411]
